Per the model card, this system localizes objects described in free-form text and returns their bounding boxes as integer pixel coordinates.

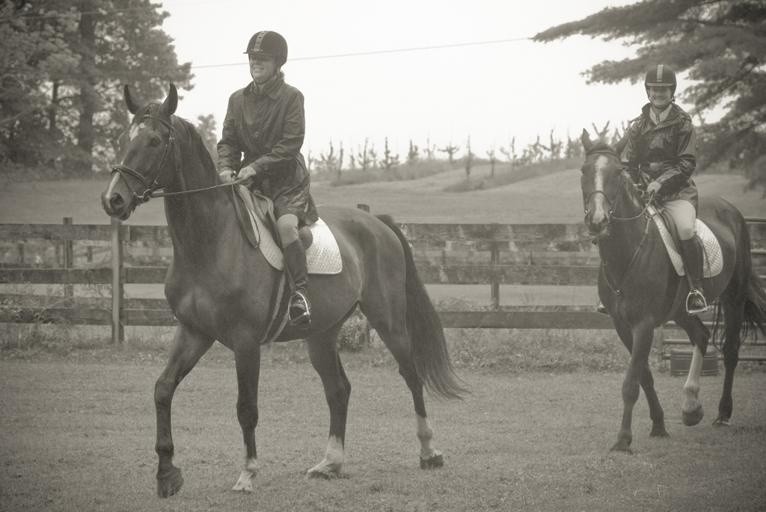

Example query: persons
[595,63,714,319]
[216,29,319,332]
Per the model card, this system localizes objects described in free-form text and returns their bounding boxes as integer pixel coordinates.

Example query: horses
[578,126,766,459]
[99,80,474,500]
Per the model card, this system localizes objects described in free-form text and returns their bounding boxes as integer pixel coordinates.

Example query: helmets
[644,64,677,96]
[242,30,288,65]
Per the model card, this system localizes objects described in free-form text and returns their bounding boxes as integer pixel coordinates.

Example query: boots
[678,233,707,310]
[281,237,312,331]
